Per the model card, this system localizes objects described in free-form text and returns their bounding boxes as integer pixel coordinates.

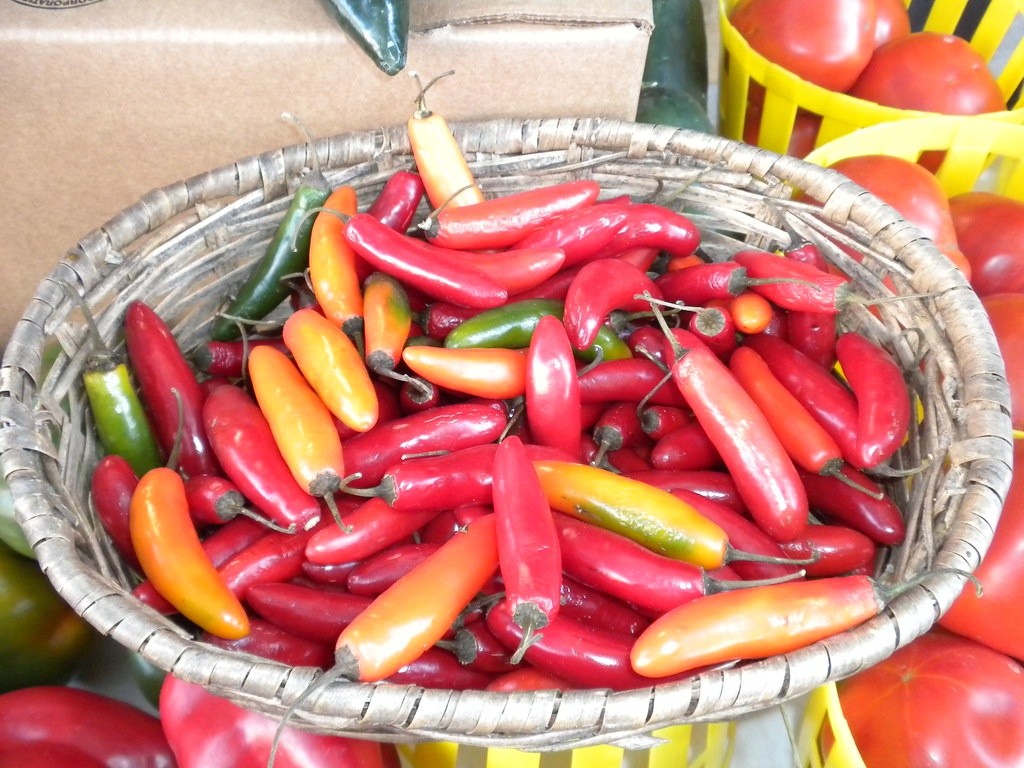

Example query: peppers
[1,519,383,768]
[74,71,935,768]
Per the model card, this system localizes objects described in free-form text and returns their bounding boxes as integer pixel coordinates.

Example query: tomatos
[725,0,1024,768]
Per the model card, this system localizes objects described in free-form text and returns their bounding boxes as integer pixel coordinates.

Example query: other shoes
[328,0,410,77]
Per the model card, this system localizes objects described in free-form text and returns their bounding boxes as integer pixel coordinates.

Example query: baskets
[0,114,1016,743]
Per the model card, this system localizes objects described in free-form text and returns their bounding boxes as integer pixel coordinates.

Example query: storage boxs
[0,0,654,366]
